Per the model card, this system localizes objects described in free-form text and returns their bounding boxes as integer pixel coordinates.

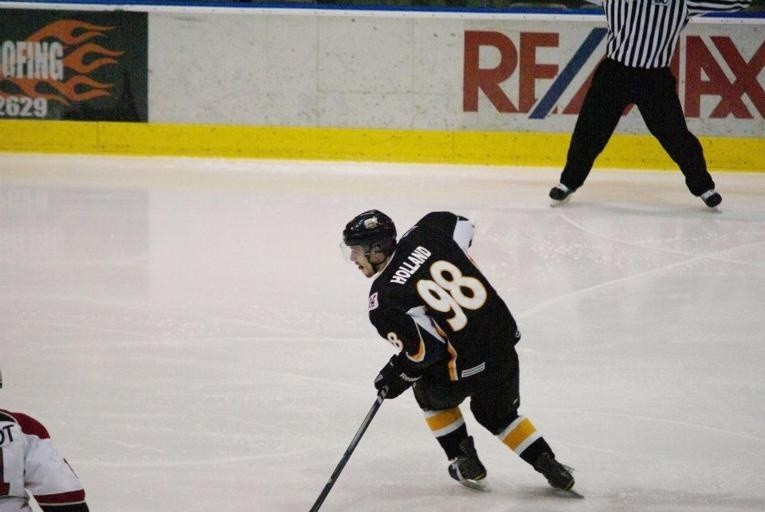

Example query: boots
[531,452,575,491]
[448,435,487,480]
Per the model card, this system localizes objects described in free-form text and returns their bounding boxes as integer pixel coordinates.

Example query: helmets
[343,209,397,246]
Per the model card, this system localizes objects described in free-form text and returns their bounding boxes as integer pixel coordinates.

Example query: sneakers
[699,188,722,208]
[550,181,575,200]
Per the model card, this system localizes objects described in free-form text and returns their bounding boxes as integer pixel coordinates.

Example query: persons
[0,405,90,511]
[339,208,578,493]
[546,0,750,206]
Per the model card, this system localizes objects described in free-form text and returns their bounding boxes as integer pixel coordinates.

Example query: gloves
[373,354,425,399]
